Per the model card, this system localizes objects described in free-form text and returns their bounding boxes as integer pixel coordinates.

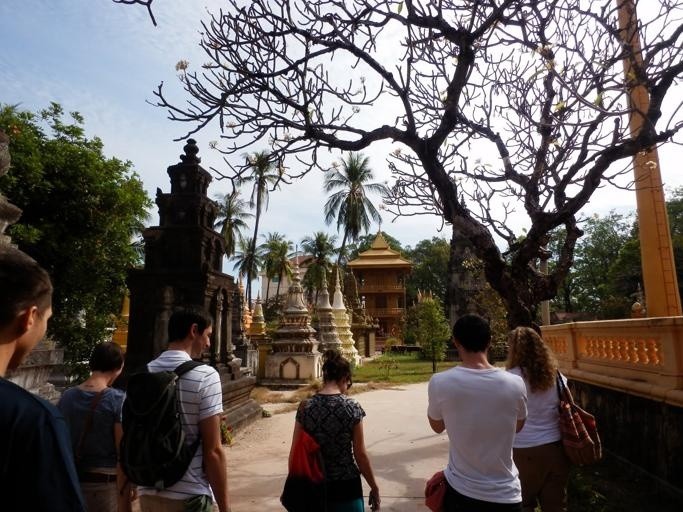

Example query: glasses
[346,375,352,391]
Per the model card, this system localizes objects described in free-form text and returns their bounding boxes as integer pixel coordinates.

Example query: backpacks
[120,358,205,491]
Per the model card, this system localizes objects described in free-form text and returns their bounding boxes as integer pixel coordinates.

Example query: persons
[427,314,529,512]
[505,327,572,512]
[57,341,127,512]
[0,242,80,512]
[136,303,231,512]
[289,349,381,512]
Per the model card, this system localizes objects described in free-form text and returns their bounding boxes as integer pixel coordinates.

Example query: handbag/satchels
[555,365,604,470]
[424,470,448,512]
[280,399,327,512]
